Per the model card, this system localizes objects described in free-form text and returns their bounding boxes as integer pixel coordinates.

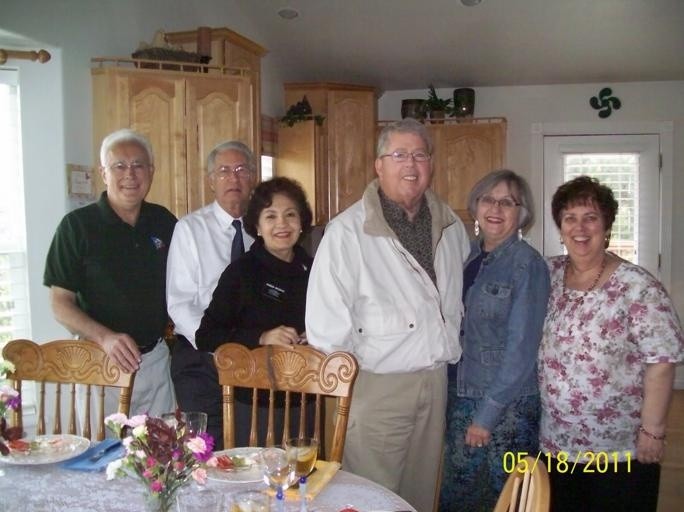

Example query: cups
[453,88,474,118]
[400,99,426,119]
[183,410,207,434]
[221,489,272,512]
[174,485,222,511]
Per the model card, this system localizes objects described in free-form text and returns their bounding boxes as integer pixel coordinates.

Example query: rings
[477,443,482,448]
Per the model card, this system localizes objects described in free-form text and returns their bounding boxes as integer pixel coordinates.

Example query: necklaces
[563,254,608,302]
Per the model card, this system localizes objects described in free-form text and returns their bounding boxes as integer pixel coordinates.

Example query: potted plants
[416,82,468,126]
[126,30,213,77]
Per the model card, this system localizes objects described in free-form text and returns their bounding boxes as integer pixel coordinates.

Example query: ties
[231,220,244,263]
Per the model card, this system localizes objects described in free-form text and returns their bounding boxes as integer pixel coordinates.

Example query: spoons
[287,467,317,488]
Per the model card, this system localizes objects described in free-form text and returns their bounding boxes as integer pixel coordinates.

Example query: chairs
[486,456,553,512]
[205,335,360,473]
[1,337,141,446]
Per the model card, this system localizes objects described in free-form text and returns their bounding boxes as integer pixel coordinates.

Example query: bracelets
[639,425,666,440]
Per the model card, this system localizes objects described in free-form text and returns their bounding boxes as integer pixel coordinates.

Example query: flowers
[101,409,217,510]
[0,355,31,459]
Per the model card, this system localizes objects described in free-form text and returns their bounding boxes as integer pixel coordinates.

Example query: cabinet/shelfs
[271,115,327,231]
[83,56,259,238]
[280,78,385,231]
[371,111,510,229]
[157,24,271,166]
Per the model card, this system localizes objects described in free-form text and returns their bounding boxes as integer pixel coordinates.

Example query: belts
[139,343,155,355]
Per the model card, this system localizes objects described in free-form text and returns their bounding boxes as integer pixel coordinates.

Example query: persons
[437,169,551,512]
[537,176,684,512]
[304,117,471,512]
[43,129,179,442]
[195,175,313,447]
[165,141,256,451]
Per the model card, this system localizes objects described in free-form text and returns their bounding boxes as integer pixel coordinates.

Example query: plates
[202,445,288,483]
[0,434,89,465]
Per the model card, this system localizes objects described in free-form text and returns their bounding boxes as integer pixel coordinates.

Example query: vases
[400,96,429,123]
[450,84,476,125]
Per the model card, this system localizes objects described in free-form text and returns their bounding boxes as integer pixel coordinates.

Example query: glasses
[379,151,431,162]
[481,194,520,209]
[210,165,252,179]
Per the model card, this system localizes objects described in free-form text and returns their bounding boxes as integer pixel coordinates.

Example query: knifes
[89,441,119,462]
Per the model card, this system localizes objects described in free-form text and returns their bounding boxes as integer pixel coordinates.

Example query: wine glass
[284,436,317,512]
[259,442,298,511]
